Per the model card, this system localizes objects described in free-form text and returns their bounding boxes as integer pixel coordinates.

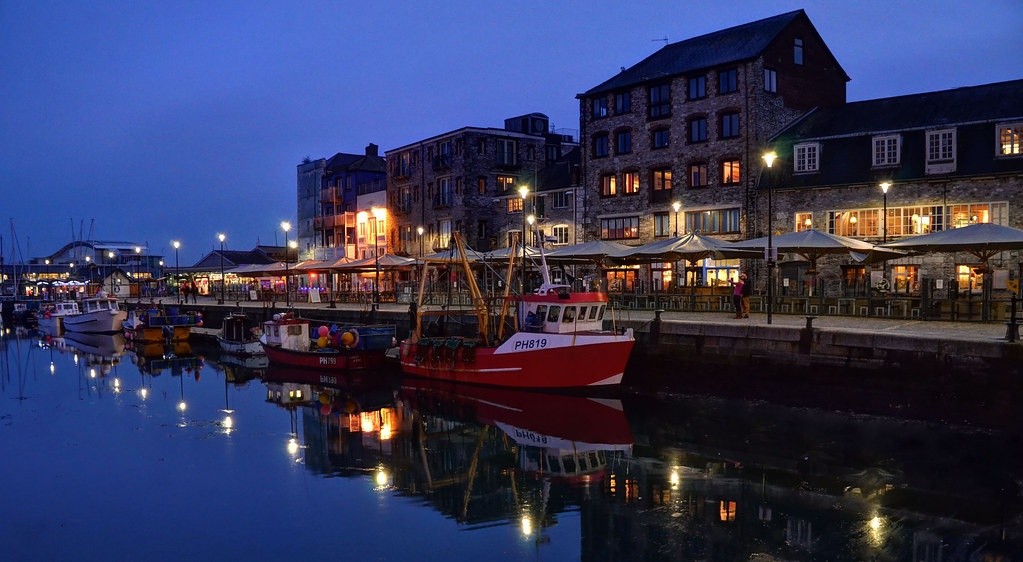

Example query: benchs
[750,302,760,311]
[810,303,831,313]
[859,304,868,315]
[629,300,647,308]
[781,302,802,312]
[878,305,899,316]
[650,301,672,309]
[723,302,734,311]
[911,307,922,317]
[829,304,850,314]
[614,299,629,308]
[660,301,687,309]
[689,301,718,310]
[765,303,782,312]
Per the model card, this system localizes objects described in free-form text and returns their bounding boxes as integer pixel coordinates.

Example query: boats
[61,290,128,335]
[121,297,201,342]
[128,341,205,400]
[259,360,398,416]
[399,376,637,550]
[35,300,82,327]
[63,331,127,389]
[258,309,404,370]
[215,351,270,387]
[35,324,68,353]
[214,308,266,355]
[396,217,634,391]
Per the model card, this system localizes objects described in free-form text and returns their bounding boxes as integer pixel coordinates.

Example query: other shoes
[733,316,741,319]
[743,316,750,318]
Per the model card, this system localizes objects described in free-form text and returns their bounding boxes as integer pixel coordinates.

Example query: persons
[728,278,745,318]
[180,281,189,303]
[191,282,198,303]
[737,273,751,319]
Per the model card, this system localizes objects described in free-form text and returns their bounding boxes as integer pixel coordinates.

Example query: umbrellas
[608,231,740,294]
[337,254,411,299]
[402,247,482,297]
[724,227,911,301]
[303,257,357,292]
[527,238,636,293]
[224,258,319,292]
[473,246,548,298]
[871,219,1023,320]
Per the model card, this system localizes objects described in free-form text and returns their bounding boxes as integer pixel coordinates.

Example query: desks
[609,292,923,318]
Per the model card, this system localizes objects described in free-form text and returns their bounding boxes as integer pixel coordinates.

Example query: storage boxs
[358,327,394,351]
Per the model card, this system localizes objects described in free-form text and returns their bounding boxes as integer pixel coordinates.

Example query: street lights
[45,259,50,285]
[85,255,90,297]
[177,368,187,411]
[114,365,118,386]
[760,152,779,323]
[108,251,114,297]
[284,222,292,307]
[672,202,683,288]
[374,414,385,485]
[140,372,145,397]
[49,347,54,375]
[174,241,180,303]
[518,185,531,299]
[70,263,75,274]
[417,227,425,261]
[218,233,226,304]
[373,204,385,309]
[136,246,141,302]
[878,183,892,280]
[222,368,233,429]
[526,214,536,245]
[158,261,163,279]
[287,411,297,455]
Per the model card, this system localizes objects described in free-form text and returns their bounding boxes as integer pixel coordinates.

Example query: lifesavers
[349,328,359,348]
[114,285,120,292]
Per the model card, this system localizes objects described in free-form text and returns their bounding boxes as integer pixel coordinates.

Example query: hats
[740,273,747,279]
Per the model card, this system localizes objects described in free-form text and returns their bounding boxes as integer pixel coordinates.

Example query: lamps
[329,324,340,336]
[986,268,994,274]
[558,291,571,300]
[804,272,820,276]
[973,268,984,274]
